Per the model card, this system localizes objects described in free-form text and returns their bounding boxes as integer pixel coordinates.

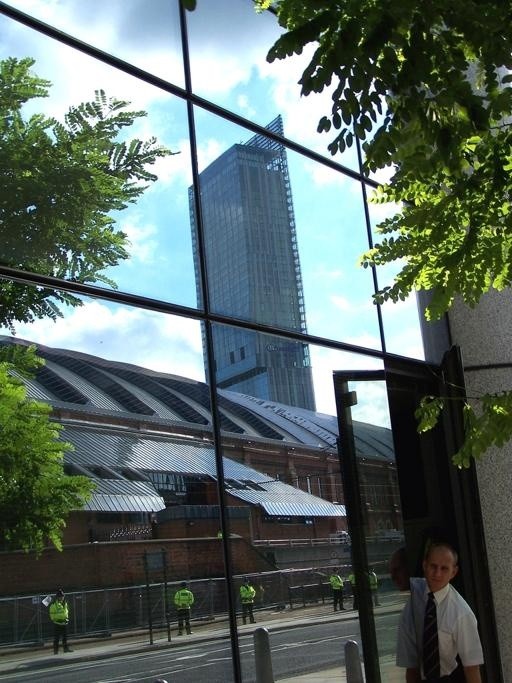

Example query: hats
[56,588,65,597]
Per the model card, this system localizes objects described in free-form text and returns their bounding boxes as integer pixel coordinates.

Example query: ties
[422,592,441,681]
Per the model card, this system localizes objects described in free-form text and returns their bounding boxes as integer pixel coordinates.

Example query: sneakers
[176,629,195,635]
[54,649,75,655]
[333,604,381,612]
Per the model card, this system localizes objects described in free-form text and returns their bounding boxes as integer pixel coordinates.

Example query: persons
[239,577,257,624]
[339,530,348,541]
[395,544,484,682]
[217,529,223,538]
[348,569,360,611]
[49,588,75,654]
[330,566,347,612]
[174,581,195,636]
[368,566,382,607]
